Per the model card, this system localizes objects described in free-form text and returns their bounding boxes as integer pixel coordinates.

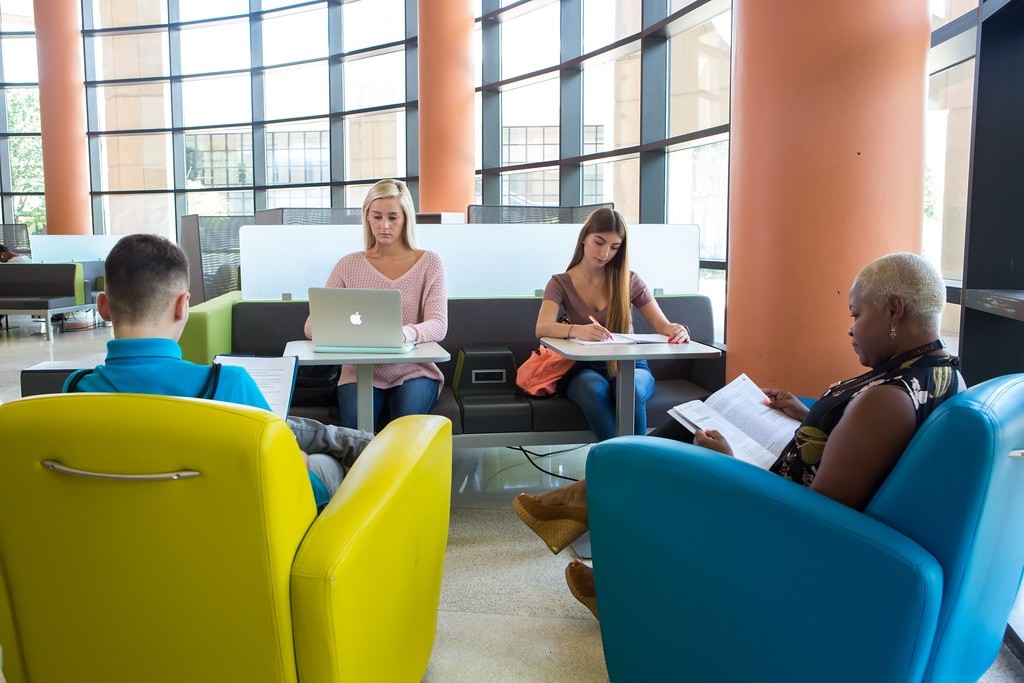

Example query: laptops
[308,287,417,353]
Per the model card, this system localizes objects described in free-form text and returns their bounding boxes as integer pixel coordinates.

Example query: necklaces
[577,264,606,292]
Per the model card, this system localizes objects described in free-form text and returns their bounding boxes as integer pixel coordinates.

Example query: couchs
[1,391,456,682]
[0,262,86,341]
[182,289,728,453]
[583,372,1023,682]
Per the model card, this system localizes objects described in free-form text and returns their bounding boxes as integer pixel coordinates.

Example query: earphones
[1,255,4,259]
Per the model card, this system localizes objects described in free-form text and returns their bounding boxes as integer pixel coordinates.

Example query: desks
[280,339,452,436]
[539,333,722,436]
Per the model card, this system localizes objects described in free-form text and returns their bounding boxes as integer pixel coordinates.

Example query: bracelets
[568,323,575,341]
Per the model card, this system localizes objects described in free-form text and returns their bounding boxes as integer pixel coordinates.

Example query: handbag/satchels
[516,319,579,400]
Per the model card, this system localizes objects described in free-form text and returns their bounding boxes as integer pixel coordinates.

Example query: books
[667,372,801,471]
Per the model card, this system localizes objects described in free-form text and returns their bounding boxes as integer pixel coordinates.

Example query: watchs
[682,324,690,336]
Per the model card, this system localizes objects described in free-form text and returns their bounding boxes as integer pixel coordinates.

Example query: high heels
[512,492,589,556]
[565,561,600,623]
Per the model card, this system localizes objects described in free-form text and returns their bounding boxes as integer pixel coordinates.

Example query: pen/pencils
[587,314,614,342]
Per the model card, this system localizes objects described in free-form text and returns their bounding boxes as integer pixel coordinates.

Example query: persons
[0,244,32,331]
[60,233,345,521]
[536,207,655,442]
[304,180,449,430]
[511,252,970,621]
